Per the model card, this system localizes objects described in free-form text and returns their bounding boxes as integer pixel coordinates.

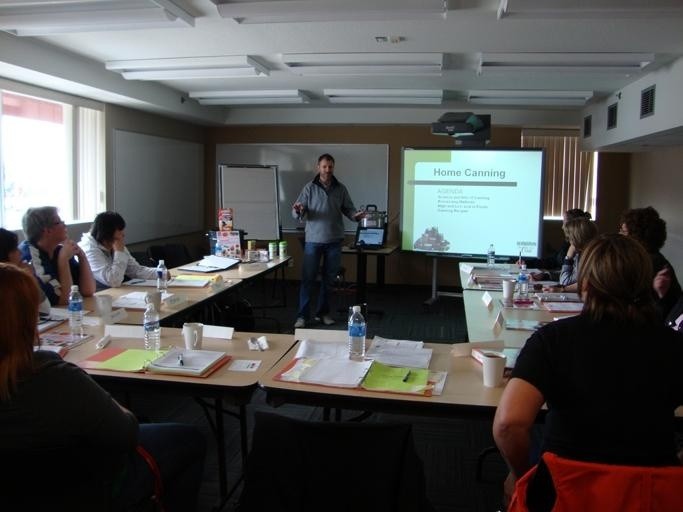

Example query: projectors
[431,122,476,136]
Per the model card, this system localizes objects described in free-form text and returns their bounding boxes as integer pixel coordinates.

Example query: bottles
[487,244,495,267]
[156,260,168,293]
[143,304,161,350]
[348,306,366,361]
[68,285,84,329]
[215,240,222,257]
[517,270,528,299]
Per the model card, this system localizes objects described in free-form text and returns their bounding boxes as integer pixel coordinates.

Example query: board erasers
[296,227,304,230]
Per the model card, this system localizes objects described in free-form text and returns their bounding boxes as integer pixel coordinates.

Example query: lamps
[324,88,444,104]
[476,53,655,77]
[469,92,593,106]
[0,0,196,36]
[189,90,312,107]
[280,53,444,78]
[105,56,270,81]
[208,0,447,23]
[497,0,683,20]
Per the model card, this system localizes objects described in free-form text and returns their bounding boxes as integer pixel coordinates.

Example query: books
[534,292,585,313]
[275,357,444,397]
[74,346,232,378]
[178,254,240,273]
[36,308,91,333]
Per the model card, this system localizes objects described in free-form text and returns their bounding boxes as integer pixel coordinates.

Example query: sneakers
[315,314,336,325]
[294,317,307,328]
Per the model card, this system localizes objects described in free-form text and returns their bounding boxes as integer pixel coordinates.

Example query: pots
[358,205,387,229]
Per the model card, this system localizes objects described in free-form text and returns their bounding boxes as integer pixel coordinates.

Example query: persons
[514,205,682,334]
[291,153,368,328]
[17,207,95,305]
[491,232,682,503]
[0,262,205,511]
[0,227,51,320]
[73,210,170,292]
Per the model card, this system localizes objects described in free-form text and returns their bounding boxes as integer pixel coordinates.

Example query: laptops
[351,227,388,249]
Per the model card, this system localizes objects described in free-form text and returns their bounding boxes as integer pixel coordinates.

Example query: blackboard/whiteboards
[214,143,391,233]
[218,165,280,242]
[112,127,205,247]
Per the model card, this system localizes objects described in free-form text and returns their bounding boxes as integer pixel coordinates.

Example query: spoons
[250,336,264,352]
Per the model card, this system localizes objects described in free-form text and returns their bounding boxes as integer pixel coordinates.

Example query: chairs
[0,437,112,511]
[510,451,683,512]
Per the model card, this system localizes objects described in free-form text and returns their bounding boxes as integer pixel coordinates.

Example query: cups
[145,290,161,312]
[522,265,526,271]
[183,323,203,350]
[269,241,288,260]
[96,295,112,320]
[503,279,513,299]
[482,351,506,388]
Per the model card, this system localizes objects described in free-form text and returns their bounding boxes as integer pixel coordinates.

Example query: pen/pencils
[131,280,145,284]
[402,370,411,381]
[519,252,521,266]
[197,263,219,268]
[178,354,183,367]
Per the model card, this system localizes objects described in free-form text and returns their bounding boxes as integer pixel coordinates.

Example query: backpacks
[222,294,255,332]
